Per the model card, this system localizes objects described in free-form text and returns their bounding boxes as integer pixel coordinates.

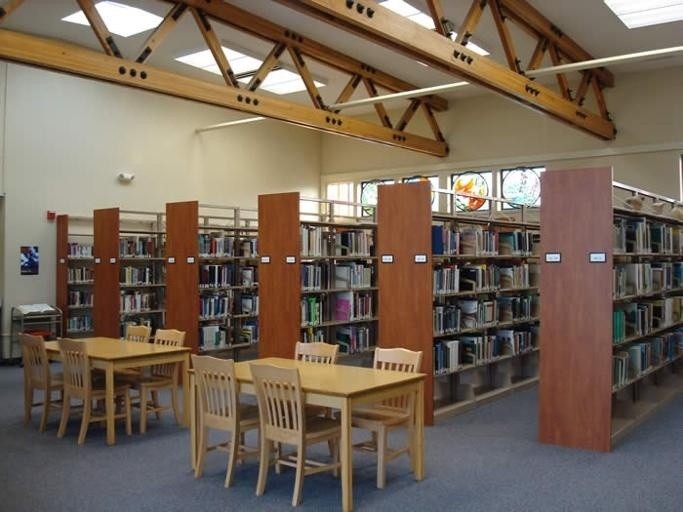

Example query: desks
[177,355,432,509]
[32,335,194,449]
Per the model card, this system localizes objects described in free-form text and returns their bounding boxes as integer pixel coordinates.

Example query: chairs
[11,329,71,439]
[55,337,130,445]
[282,339,353,481]
[333,344,432,490]
[245,358,348,507]
[184,352,290,486]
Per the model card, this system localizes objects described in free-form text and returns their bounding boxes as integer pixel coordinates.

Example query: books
[431,224,542,373]
[198,233,260,348]
[612,218,683,387]
[300,225,377,354]
[68,244,94,330]
[15,302,56,315]
[120,236,167,337]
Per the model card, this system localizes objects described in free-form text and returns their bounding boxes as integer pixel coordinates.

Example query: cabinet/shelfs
[6,303,64,354]
[164,199,258,391]
[256,186,378,368]
[376,183,540,430]
[55,206,167,342]
[535,167,681,455]
